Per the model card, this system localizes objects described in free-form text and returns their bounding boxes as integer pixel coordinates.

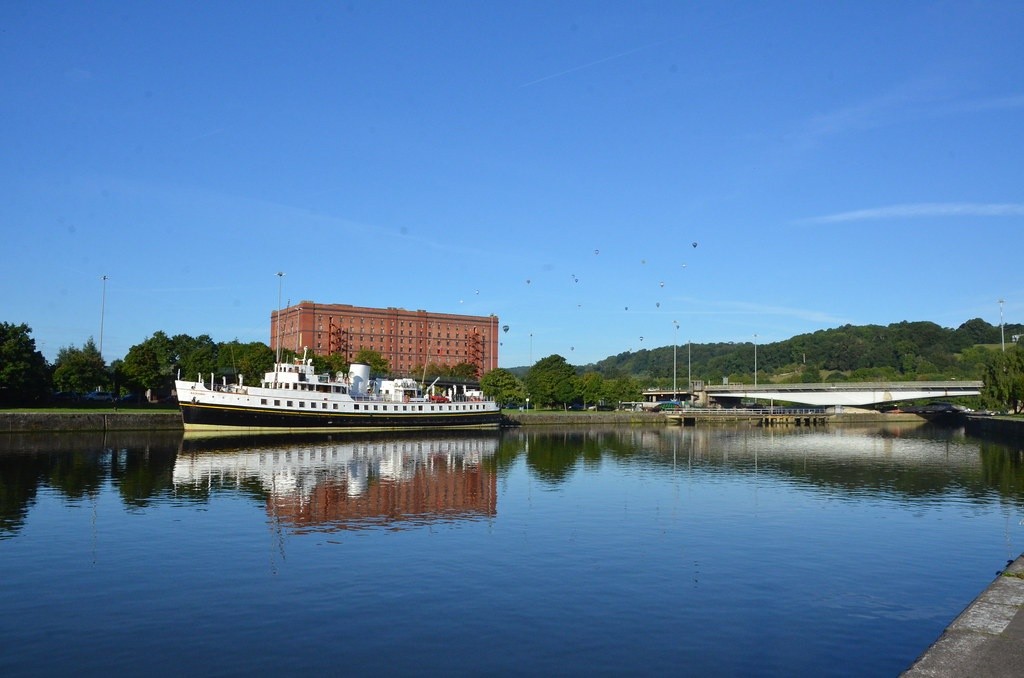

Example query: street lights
[672,319,681,401]
[753,334,759,388]
[275,272,286,363]
[98,275,111,355]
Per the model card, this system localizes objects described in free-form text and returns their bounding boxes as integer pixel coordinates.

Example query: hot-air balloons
[459,238,700,354]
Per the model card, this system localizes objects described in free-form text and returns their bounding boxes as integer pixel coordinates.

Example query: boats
[177,346,504,432]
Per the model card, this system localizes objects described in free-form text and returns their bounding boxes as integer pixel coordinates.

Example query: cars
[55,392,75,403]
[88,392,113,403]
[502,402,518,410]
[651,403,680,412]
[568,403,584,410]
